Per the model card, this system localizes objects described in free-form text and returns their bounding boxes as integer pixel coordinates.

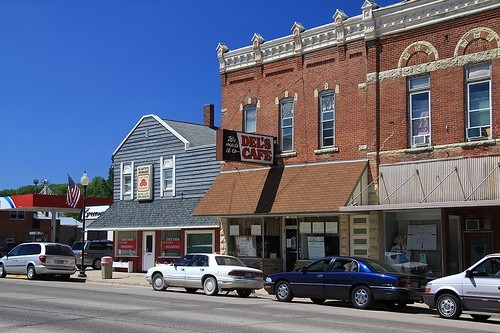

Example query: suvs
[71,239,113,269]
[423,253,500,321]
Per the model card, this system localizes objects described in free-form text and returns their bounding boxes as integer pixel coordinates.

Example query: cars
[263,256,423,309]
[0,243,77,280]
[385,252,432,278]
[144,252,262,298]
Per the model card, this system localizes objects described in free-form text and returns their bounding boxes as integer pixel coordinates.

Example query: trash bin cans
[100,256,113,279]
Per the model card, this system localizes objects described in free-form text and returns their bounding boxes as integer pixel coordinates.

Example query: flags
[66,177,81,208]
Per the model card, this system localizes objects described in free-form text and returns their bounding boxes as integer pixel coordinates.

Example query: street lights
[78,170,89,277]
